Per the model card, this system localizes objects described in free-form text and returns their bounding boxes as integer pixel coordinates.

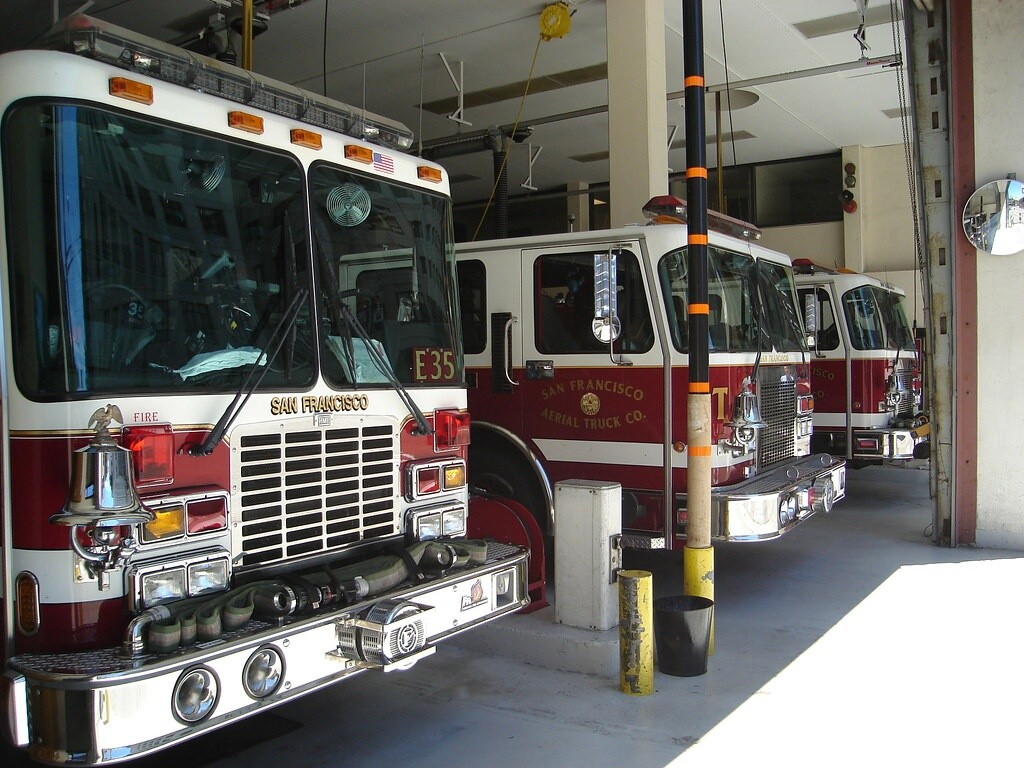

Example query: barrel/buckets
[653,595,714,677]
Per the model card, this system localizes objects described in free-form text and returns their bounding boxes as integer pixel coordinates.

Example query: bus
[0,13,532,768]
[332,189,849,549]
[789,255,933,466]
